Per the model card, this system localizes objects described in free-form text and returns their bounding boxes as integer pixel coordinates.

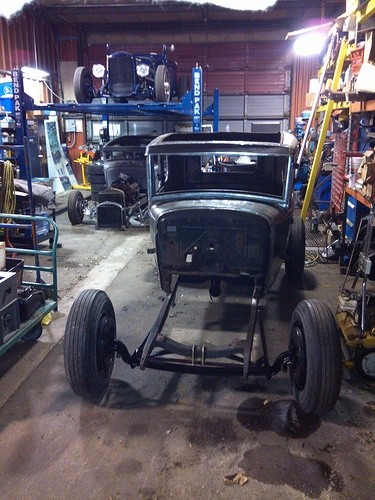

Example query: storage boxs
[0,241,46,344]
[305,78,321,109]
